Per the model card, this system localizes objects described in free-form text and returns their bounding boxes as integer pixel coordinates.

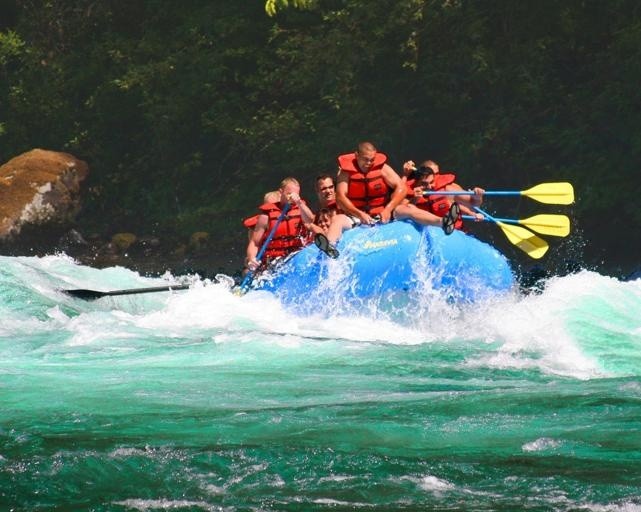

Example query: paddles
[473,206,549,259]
[460,215,570,237]
[61,285,189,303]
[421,182,574,205]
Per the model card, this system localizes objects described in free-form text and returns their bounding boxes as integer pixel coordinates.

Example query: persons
[237,177,316,285]
[313,206,332,231]
[243,190,326,247]
[313,174,339,210]
[389,165,485,228]
[313,141,460,261]
[400,159,484,224]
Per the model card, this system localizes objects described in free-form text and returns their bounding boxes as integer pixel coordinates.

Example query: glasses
[320,185,333,190]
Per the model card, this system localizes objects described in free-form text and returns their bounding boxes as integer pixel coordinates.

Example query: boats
[243,216,520,322]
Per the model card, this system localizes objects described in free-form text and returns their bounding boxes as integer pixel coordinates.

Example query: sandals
[313,232,339,259]
[442,201,459,235]
